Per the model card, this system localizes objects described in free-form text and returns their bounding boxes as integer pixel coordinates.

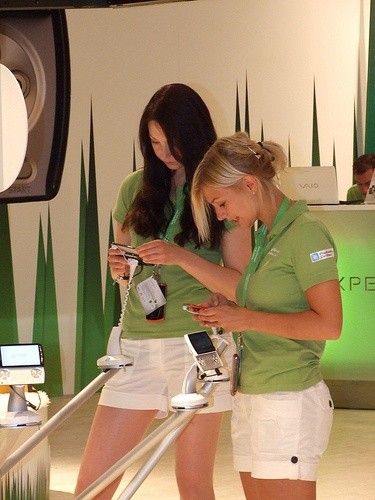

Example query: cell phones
[230,354,240,396]
[0,343,44,368]
[184,331,216,356]
[146,284,168,322]
[183,305,208,314]
[112,242,144,262]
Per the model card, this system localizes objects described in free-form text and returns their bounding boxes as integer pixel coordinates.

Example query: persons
[72,82,253,500]
[347,154,375,204]
[190,130,344,499]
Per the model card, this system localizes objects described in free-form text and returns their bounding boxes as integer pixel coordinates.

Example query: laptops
[275,166,365,206]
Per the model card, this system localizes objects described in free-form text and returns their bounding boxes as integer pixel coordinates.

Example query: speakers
[0,8,71,204]
